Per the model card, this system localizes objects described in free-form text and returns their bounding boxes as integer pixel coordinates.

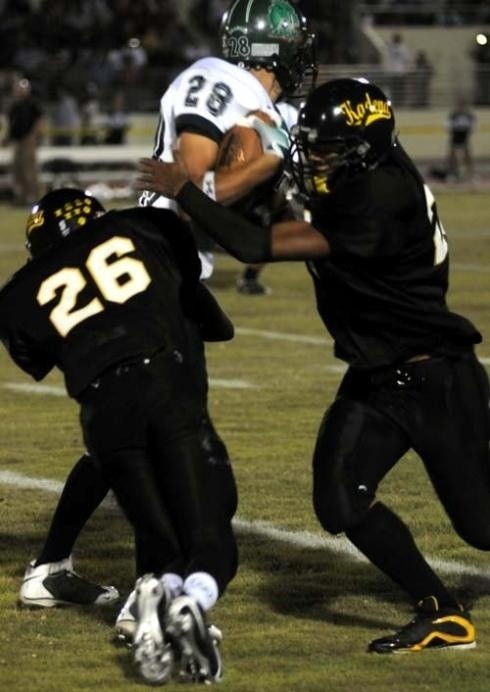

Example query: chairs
[115,590,142,649]
[18,555,118,611]
[367,592,479,659]
[128,571,175,689]
[164,594,224,684]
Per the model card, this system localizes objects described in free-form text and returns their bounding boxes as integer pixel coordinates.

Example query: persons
[386,31,433,108]
[0,185,241,687]
[16,0,320,608]
[0,0,221,201]
[436,0,489,105]
[445,98,478,183]
[131,73,490,656]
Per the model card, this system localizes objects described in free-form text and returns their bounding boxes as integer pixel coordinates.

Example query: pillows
[218,0,313,92]
[24,188,107,260]
[287,75,396,171]
